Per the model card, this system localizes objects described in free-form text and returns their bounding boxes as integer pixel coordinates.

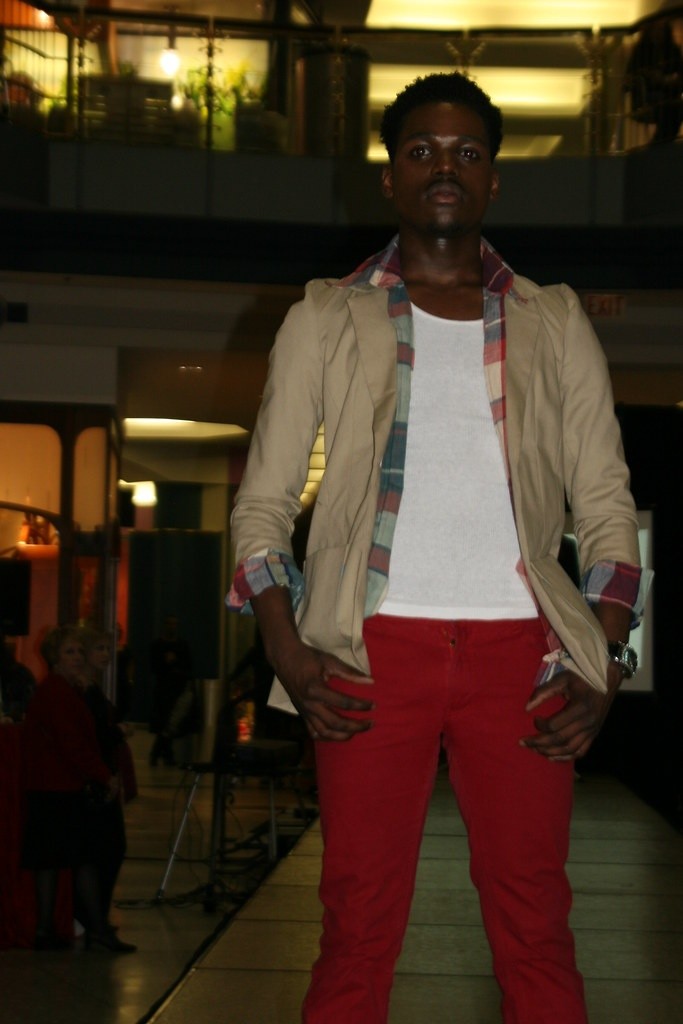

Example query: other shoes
[86,925,137,955]
[33,935,75,951]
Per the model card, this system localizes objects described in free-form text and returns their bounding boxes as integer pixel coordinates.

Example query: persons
[148,614,191,769]
[115,629,135,735]
[621,17,683,144]
[19,625,138,955]
[228,71,644,1024]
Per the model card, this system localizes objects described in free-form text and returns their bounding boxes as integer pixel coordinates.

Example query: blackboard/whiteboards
[114,525,225,725]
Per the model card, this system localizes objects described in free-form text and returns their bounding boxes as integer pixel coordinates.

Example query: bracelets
[607,640,637,680]
[128,679,133,682]
